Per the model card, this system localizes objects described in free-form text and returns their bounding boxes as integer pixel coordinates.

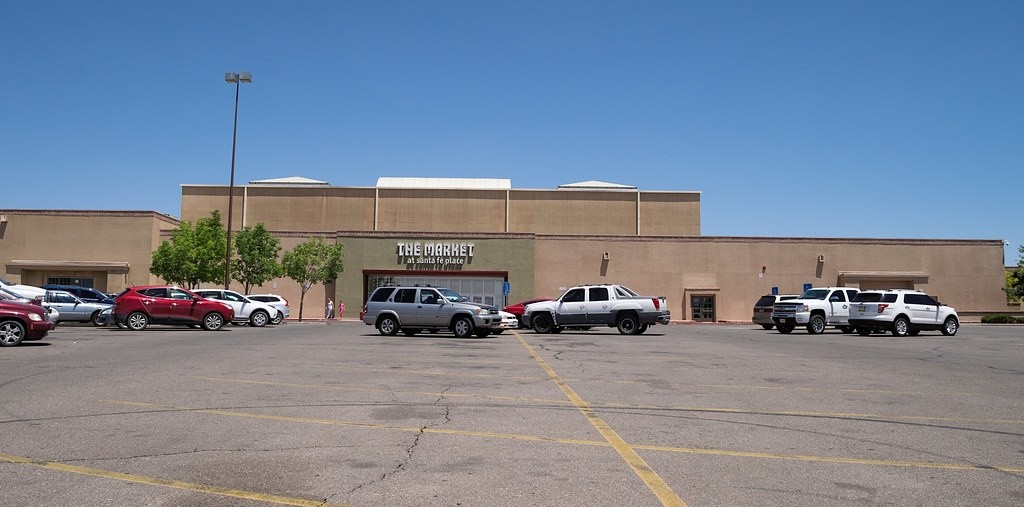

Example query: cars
[503,297,555,330]
[98,304,129,330]
[0,300,55,347]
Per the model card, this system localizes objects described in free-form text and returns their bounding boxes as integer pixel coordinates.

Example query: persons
[338,299,345,317]
[326,297,334,319]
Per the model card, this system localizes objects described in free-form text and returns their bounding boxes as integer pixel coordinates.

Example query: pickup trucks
[521,284,671,335]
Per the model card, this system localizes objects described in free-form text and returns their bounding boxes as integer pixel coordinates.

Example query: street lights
[224,70,254,290]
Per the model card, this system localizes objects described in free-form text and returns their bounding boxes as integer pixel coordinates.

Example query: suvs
[359,286,519,338]
[184,288,278,329]
[0,279,112,327]
[171,288,289,327]
[848,289,960,336]
[771,287,887,336]
[114,284,235,331]
[751,293,802,331]
